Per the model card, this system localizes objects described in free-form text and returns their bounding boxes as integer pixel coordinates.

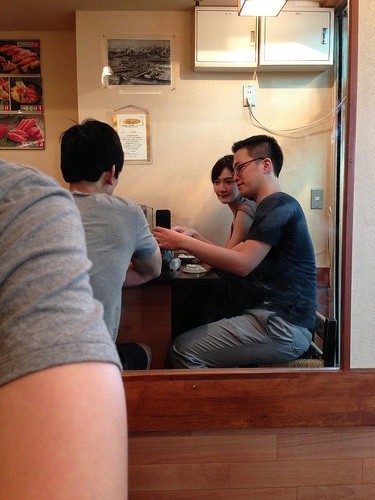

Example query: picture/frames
[101,34,175,91]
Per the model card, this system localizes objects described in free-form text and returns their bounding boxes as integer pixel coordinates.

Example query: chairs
[252,310,336,367]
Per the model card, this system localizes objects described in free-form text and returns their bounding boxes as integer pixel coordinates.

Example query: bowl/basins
[10,82,42,108]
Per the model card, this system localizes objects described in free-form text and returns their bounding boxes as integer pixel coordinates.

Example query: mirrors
[0,0,358,369]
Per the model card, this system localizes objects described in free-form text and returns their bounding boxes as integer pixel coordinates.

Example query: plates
[183,265,206,274]
[178,255,195,259]
[6,124,44,147]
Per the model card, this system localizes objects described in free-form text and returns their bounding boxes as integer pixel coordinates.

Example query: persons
[59,118,162,371]
[153,135,318,369]
[0,160,128,500]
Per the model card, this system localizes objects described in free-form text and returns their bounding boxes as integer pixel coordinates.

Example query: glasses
[230,157,266,175]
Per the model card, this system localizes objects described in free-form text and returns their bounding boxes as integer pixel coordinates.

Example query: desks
[118,249,219,369]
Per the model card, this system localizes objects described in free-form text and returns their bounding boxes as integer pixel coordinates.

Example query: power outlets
[244,84,256,108]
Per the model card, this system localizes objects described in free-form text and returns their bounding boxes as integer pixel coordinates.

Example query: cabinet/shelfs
[194,6,333,74]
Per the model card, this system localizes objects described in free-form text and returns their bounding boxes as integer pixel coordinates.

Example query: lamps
[237,0,288,17]
